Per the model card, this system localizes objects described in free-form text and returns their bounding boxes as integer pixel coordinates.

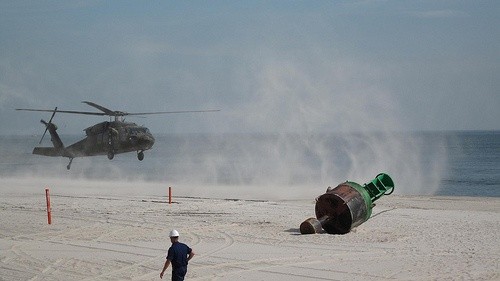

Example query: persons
[160,229,195,281]
[107,125,119,146]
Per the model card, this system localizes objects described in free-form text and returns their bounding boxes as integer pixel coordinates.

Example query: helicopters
[14,99,223,170]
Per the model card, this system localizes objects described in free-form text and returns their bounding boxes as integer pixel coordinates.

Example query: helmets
[169,229,180,237]
